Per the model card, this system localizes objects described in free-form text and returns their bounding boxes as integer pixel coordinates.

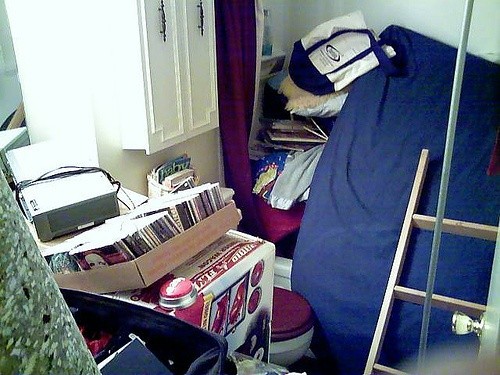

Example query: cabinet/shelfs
[110,0,220,156]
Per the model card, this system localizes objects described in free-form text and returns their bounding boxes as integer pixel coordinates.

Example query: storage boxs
[51,199,239,295]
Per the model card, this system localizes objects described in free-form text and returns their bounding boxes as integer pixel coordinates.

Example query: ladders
[363,148,499,375]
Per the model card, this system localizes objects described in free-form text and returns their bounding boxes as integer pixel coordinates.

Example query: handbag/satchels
[288,8,398,96]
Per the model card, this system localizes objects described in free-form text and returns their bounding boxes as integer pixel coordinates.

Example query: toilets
[269,285,316,367]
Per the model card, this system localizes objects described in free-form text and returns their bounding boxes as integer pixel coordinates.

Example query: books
[148,152,196,186]
[43,179,228,277]
[262,117,329,153]
